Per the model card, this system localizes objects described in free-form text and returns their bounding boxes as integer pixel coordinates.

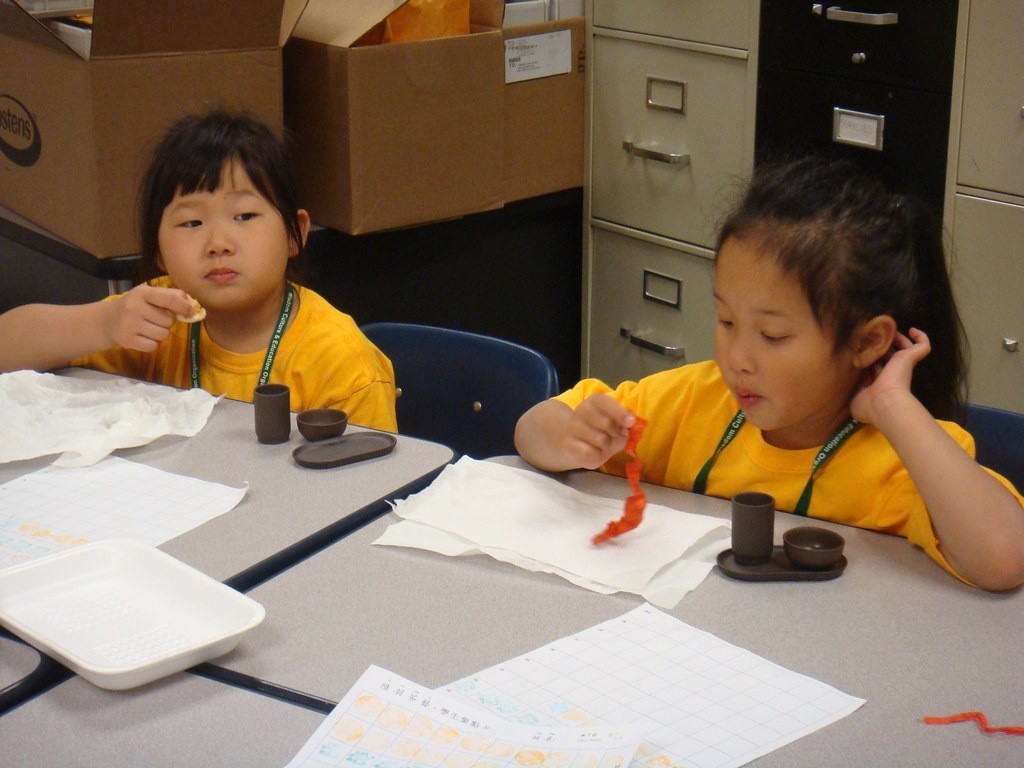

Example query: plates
[717,546,847,582]
[0,537,267,690]
[293,432,397,469]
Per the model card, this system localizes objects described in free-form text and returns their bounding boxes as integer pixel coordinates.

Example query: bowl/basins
[783,527,845,568]
[296,409,348,441]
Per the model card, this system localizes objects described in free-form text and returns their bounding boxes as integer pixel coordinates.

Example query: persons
[0,109,398,432]
[514,154,1024,594]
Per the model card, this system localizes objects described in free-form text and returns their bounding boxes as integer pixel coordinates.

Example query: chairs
[327,322,558,460]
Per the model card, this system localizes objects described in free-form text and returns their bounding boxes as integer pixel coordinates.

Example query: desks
[0,671,331,768]
[0,635,42,700]
[187,455,1024,768]
[0,364,455,590]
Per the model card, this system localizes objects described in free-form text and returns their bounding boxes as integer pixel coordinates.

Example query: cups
[255,384,289,444]
[732,492,774,562]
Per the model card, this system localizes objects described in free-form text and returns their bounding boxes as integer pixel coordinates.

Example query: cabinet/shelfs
[582,0,1024,414]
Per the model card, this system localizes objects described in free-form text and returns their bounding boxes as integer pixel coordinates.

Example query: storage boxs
[288,0,585,236]
[0,0,310,261]
